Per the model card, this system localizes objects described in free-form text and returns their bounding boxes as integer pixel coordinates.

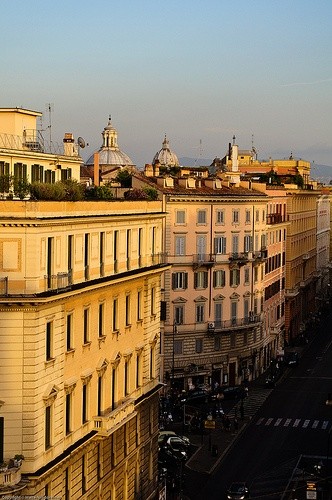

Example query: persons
[159,375,278,489]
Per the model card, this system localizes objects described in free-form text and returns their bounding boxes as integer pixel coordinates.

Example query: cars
[158,430,190,442]
[163,435,191,452]
[287,352,299,367]
[225,480,251,500]
[179,389,214,403]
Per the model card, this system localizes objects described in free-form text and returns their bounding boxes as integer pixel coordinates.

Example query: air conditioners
[208,323,215,329]
[250,311,254,316]
[249,316,255,321]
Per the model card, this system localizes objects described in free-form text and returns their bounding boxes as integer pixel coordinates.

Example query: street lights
[240,385,248,418]
[207,410,213,451]
